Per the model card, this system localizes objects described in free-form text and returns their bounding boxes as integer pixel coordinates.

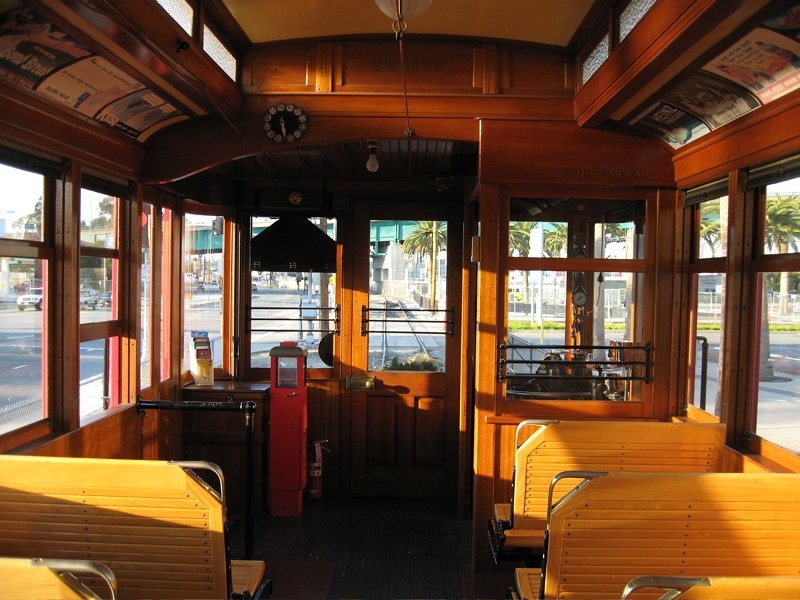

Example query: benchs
[487,422,800,600]
[0,553,118,600]
[0,454,272,600]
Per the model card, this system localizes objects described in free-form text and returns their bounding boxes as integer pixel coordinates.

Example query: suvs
[18,288,43,311]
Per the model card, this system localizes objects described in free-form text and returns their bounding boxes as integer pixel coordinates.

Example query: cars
[212,280,218,285]
[198,281,205,291]
[14,280,41,290]
[252,284,258,291]
[80,289,111,311]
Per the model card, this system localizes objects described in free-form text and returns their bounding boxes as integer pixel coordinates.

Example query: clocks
[263,103,308,145]
[572,290,587,306]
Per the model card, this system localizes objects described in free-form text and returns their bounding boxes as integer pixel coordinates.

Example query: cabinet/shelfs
[180,378,271,510]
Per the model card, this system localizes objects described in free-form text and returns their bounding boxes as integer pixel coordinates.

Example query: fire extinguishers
[309,439,330,500]
